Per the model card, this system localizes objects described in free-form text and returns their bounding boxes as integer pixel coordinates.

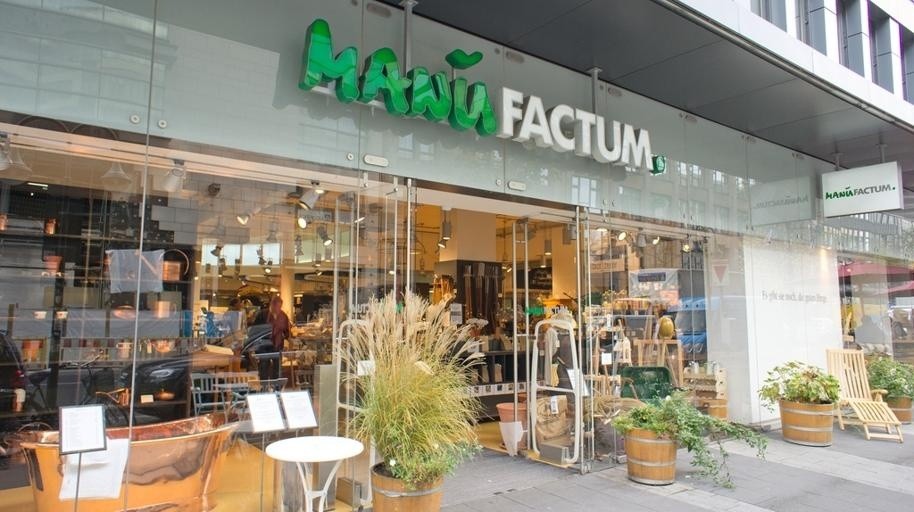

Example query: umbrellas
[839,260,914,315]
[873,280,914,297]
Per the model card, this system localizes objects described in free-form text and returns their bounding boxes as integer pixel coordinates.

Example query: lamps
[205,184,696,286]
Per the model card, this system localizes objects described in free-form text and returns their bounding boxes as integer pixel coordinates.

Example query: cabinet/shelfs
[0,175,195,470]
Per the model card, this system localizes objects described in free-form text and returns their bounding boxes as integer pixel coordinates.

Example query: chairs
[827,348,904,441]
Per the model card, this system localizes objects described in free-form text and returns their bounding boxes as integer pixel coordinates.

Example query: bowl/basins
[33,311,48,319]
[56,311,68,319]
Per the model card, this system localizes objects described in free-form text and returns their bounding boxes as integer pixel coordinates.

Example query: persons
[220,298,248,349]
[206,311,222,338]
[250,294,297,391]
[881,314,908,339]
[848,315,886,348]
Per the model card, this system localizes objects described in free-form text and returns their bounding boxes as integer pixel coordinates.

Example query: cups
[117,342,132,360]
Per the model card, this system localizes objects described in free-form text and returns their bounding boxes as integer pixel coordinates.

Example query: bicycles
[0,348,139,433]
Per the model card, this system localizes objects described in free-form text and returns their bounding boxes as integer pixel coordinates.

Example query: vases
[496,402,527,449]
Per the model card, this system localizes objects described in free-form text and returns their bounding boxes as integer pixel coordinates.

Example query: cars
[0,329,28,428]
[117,320,286,421]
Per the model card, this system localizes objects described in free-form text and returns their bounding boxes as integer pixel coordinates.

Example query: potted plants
[333,290,494,512]
[757,361,840,448]
[589,388,768,492]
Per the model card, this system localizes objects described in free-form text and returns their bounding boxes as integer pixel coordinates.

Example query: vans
[581,266,751,368]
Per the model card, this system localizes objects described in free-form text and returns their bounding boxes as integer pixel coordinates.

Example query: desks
[265,437,364,512]
